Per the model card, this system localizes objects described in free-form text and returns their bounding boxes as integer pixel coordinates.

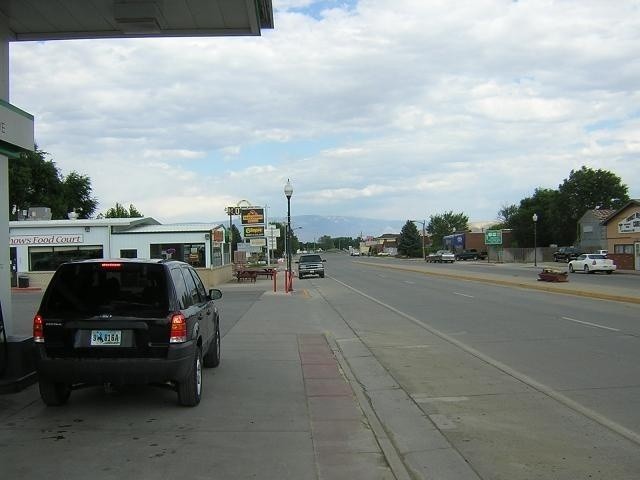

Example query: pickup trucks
[455,247,488,261]
[552,247,584,263]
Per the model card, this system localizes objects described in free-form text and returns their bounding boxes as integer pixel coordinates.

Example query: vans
[32,258,222,408]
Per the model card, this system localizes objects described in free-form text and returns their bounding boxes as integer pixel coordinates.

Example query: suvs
[295,254,326,279]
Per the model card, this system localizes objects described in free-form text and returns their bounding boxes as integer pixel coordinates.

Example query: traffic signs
[484,229,502,245]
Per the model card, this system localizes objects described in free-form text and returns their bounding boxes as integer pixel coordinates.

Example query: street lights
[283,178,294,291]
[409,219,426,260]
[452,225,456,255]
[286,226,304,271]
[531,213,539,267]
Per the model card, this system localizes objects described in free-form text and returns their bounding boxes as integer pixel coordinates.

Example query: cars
[424,249,456,264]
[567,253,617,274]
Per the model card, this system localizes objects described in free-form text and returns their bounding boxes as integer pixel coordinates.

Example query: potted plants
[538,268,568,282]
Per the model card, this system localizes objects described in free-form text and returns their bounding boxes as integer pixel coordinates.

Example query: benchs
[236,268,273,283]
